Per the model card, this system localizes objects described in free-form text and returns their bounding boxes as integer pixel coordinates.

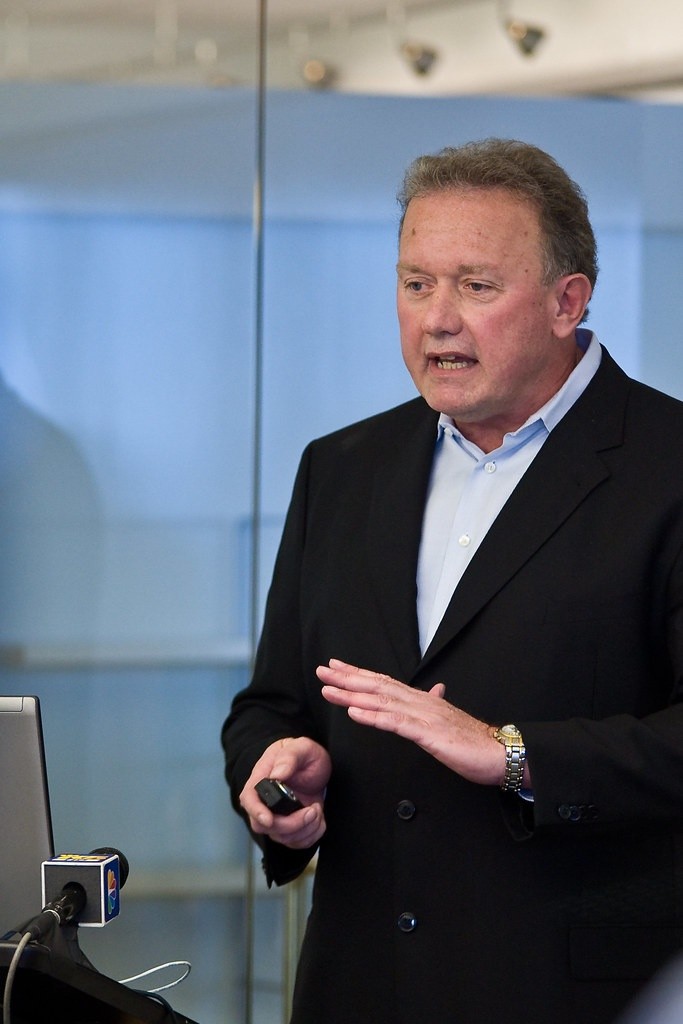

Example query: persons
[220,141,683,1024]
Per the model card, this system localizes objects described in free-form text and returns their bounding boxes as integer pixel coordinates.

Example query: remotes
[254,778,305,815]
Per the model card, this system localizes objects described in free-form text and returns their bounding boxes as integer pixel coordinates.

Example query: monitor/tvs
[0,695,55,939]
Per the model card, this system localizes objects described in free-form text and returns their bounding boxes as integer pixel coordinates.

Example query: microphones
[21,847,129,940]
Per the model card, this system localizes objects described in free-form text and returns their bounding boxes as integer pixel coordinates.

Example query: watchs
[494,724,526,791]
